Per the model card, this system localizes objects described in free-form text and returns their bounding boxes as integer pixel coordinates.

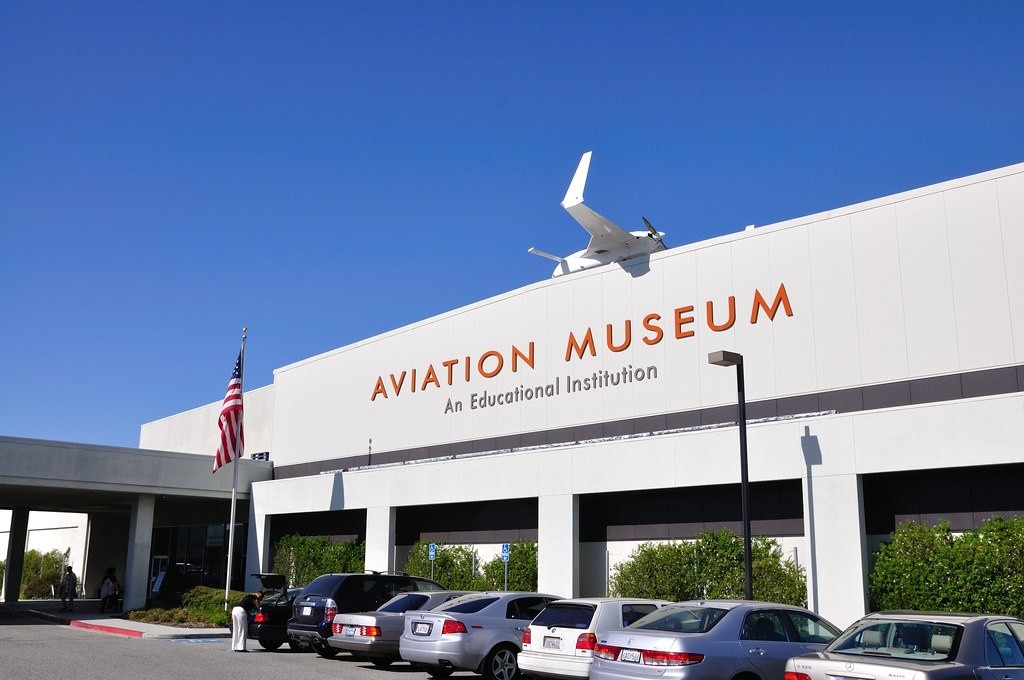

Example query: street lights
[706,348,756,600]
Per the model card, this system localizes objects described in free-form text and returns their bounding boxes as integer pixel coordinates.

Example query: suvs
[285,569,449,659]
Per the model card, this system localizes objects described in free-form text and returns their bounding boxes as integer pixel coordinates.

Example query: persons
[230,591,264,653]
[99,566,120,614]
[59,566,78,612]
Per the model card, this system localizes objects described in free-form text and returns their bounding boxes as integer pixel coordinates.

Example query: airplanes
[528,152,668,278]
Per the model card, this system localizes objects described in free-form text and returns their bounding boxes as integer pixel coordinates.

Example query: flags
[212,350,245,475]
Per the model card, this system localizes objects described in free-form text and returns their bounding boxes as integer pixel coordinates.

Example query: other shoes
[60,607,67,611]
[235,650,250,652]
[65,609,72,613]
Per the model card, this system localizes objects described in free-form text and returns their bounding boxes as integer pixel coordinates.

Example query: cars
[785,607,1023,679]
[516,597,675,680]
[589,601,841,680]
[398,590,566,680]
[326,590,480,667]
[230,572,303,650]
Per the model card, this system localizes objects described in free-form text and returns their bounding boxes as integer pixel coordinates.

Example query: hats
[105,567,116,573]
